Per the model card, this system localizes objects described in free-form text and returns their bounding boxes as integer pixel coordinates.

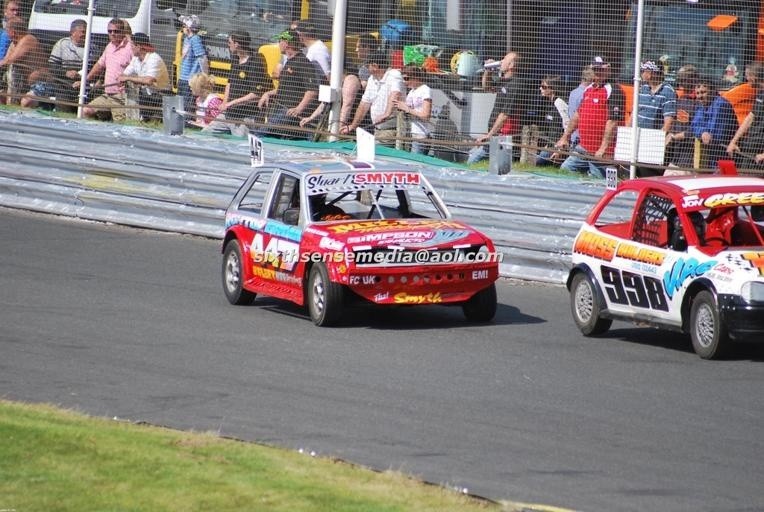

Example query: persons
[535,55,626,178]
[291,180,351,226]
[252,2,291,22]
[1,2,174,122]
[466,51,525,166]
[201,30,266,138]
[626,59,763,177]
[254,20,331,143]
[668,212,706,252]
[300,35,432,155]
[176,14,208,129]
[185,72,224,128]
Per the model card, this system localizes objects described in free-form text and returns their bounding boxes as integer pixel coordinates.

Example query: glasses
[542,86,547,89]
[404,77,409,81]
[108,30,120,33]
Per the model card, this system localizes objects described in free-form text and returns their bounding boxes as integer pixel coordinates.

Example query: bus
[25,0,764,158]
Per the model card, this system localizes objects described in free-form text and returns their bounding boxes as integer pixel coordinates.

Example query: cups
[396,96,403,112]
[483,61,501,67]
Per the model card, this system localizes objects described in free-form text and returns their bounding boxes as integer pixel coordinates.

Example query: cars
[222,156,501,332]
[564,175,764,363]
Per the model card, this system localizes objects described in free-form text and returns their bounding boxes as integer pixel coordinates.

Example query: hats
[131,33,155,50]
[592,56,610,70]
[640,60,663,73]
[273,31,299,40]
[177,15,200,31]
